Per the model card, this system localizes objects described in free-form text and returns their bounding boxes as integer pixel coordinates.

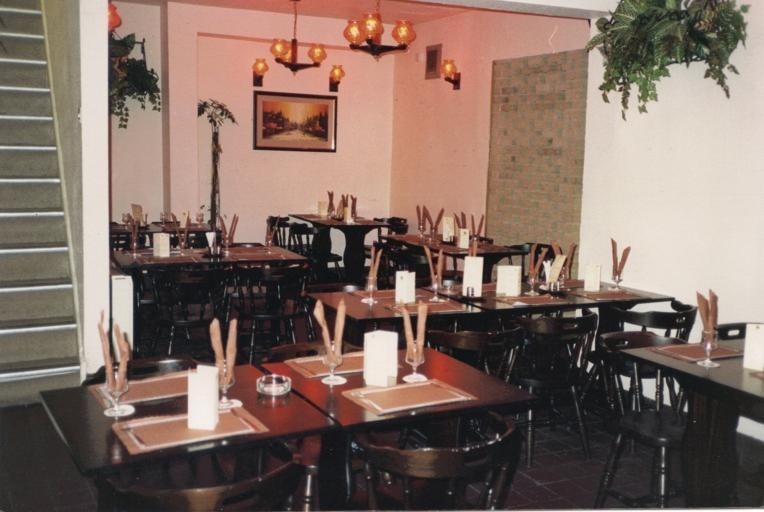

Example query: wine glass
[523,266,571,297]
[214,360,236,408]
[102,367,136,418]
[402,341,428,383]
[360,276,378,304]
[428,278,455,303]
[123,213,275,258]
[697,330,721,368]
[608,272,624,291]
[416,224,480,250]
[365,302,374,319]
[321,341,347,385]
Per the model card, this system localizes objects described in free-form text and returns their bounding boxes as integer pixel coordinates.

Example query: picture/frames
[253,89,337,152]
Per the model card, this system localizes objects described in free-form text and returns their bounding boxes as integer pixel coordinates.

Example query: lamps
[344,0,417,63]
[441,59,460,89]
[253,58,268,85]
[329,64,344,91]
[271,0,327,75]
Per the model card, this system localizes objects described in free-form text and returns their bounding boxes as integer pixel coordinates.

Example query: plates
[331,215,344,222]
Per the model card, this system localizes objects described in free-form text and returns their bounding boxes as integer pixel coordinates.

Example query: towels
[326,191,357,215]
[743,324,764,370]
